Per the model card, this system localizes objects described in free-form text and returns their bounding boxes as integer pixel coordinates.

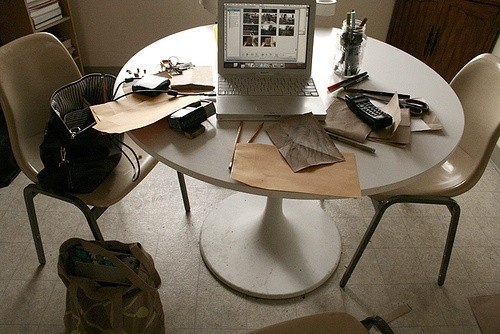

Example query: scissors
[363,93,429,116]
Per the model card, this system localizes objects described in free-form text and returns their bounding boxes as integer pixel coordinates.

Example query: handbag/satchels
[38,72,125,195]
[56,237,167,334]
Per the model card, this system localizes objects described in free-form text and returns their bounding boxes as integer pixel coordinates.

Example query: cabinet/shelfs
[20,1,84,78]
[385,1,499,81]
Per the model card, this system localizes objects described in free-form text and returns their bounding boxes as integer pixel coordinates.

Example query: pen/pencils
[327,72,371,92]
[346,12,350,26]
[351,9,356,28]
[344,75,369,90]
[326,131,376,153]
[360,18,367,26]
[346,89,410,99]
[228,120,244,170]
[246,121,264,144]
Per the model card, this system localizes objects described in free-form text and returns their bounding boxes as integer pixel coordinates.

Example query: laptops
[216,0,327,120]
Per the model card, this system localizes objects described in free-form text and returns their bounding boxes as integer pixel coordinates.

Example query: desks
[113,24,466,301]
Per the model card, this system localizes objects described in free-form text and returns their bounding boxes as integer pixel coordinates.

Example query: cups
[333,19,369,79]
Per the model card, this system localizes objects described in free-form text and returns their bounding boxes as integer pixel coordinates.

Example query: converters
[134,73,171,96]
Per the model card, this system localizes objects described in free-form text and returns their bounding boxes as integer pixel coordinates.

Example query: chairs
[340,52,500,287]
[248,312,395,333]
[1,33,190,258]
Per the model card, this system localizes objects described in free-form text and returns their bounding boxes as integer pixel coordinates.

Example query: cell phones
[345,92,392,129]
